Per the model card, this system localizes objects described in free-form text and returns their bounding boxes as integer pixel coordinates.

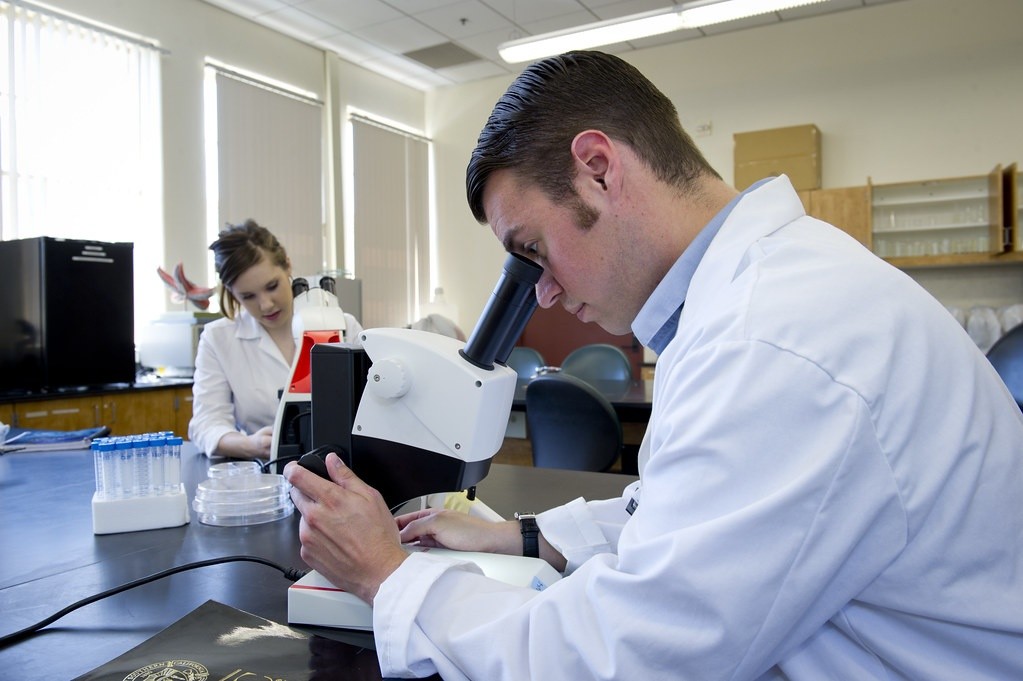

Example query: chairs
[503,342,635,471]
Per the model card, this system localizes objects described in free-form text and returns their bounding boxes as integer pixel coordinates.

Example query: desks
[512,375,654,417]
[0,435,641,681]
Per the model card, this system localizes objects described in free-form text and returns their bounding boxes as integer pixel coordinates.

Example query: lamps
[497,0,830,67]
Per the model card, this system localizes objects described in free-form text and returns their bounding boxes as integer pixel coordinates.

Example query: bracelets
[515,512,540,558]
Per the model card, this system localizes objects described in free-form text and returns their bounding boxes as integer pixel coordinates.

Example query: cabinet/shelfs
[0,377,196,443]
[731,123,1023,272]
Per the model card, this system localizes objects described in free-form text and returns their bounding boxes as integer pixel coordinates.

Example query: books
[0,428,111,452]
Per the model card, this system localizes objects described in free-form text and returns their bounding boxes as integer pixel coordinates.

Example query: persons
[188,221,365,458]
[285,52,1022,681]
